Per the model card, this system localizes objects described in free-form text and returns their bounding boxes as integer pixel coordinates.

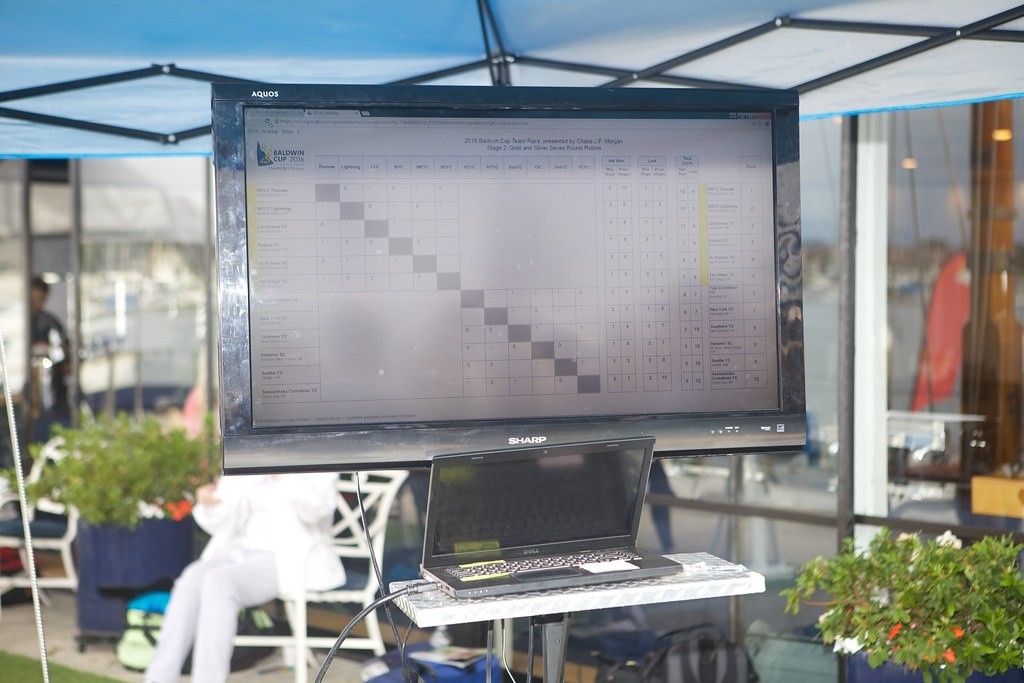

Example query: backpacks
[115,590,175,672]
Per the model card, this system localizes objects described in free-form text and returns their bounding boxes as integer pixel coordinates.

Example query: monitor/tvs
[209,80,807,474]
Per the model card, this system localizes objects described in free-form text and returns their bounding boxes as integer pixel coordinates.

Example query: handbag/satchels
[642,622,761,683]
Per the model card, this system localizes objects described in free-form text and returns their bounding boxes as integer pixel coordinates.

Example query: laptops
[419,436,681,600]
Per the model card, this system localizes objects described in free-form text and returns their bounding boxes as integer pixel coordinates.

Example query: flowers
[0,400,223,530]
[780,529,1024,683]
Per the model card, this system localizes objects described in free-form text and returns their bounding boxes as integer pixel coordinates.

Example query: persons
[144,472,345,683]
[28,278,71,466]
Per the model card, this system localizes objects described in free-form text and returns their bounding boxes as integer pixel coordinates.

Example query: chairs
[233,470,411,682]
[0,436,108,596]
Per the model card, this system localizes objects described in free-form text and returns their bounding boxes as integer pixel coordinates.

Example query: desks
[389,551,767,683]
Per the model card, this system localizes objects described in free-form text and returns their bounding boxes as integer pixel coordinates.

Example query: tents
[1,0,1024,683]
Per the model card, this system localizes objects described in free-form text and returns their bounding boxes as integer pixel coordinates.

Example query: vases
[73,519,193,633]
[848,649,1024,683]
